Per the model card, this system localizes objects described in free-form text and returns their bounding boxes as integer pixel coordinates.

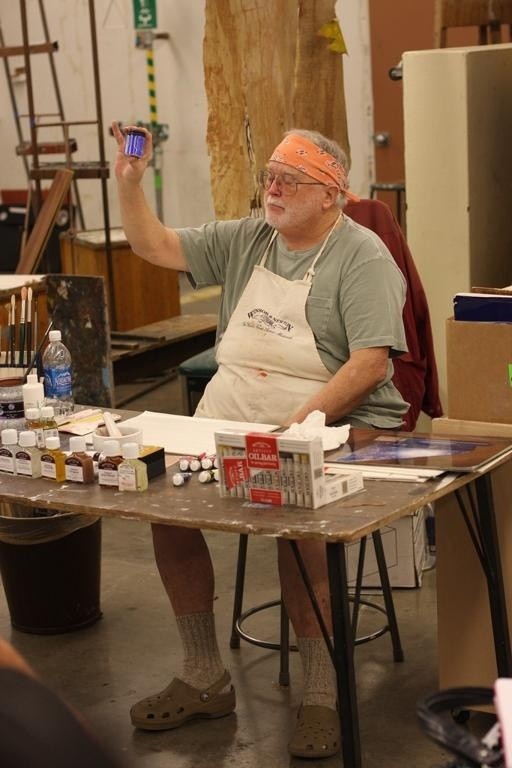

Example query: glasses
[259,170,327,198]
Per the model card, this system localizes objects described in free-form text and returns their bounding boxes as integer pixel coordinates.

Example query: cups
[92,425,144,454]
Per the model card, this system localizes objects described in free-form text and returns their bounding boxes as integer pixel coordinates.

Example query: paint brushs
[4,286,39,366]
[21,303,63,385]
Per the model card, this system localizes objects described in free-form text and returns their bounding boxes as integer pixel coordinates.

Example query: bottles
[23,407,46,450]
[124,131,146,159]
[0,428,150,493]
[21,374,45,419]
[41,407,62,451]
[42,329,75,419]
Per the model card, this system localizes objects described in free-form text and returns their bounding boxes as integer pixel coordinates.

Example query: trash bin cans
[0,502,104,635]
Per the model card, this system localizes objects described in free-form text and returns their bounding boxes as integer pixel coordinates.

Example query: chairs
[0,282,38,387]
[229,200,404,685]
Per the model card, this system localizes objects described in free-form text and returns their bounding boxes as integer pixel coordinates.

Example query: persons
[112,121,411,758]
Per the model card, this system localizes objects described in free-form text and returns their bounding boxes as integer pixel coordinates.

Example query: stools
[370,183,405,228]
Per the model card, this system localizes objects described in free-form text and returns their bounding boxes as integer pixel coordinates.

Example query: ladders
[0,0,118,329]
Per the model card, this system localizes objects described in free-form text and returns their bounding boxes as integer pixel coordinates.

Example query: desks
[1,404,512,768]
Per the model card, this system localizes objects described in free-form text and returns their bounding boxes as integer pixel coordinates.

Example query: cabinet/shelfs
[60,226,181,332]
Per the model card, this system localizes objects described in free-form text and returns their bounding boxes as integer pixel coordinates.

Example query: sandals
[130,669,236,731]
[288,696,342,759]
